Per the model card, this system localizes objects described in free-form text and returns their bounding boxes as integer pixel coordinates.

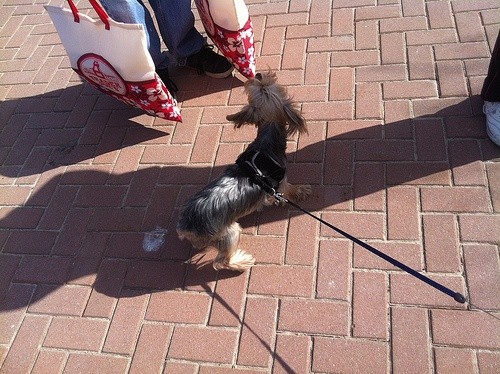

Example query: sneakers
[185,44,234,78]
[155,67,178,99]
[483,101,500,146]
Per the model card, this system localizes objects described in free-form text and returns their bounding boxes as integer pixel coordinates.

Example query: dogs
[176,72,313,272]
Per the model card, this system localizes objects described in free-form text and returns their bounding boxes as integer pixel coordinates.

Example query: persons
[481,29,500,145]
[99,0,236,102]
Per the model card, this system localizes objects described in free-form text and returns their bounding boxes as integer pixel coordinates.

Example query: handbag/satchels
[42,0,182,123]
[193,0,256,80]
